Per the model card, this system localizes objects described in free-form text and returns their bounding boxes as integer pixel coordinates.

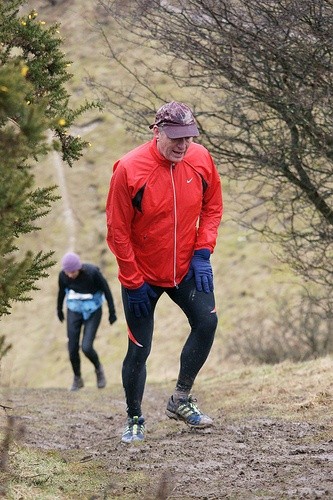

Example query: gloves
[127,282,157,317]
[58,310,63,321]
[185,248,213,293]
[109,314,116,324]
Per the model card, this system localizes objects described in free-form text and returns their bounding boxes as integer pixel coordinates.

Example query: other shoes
[71,376,84,391]
[95,365,106,388]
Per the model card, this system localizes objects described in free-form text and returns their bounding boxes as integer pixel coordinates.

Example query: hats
[62,254,81,271]
[149,101,200,138]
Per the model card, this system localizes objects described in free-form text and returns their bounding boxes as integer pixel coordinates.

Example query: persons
[57,253,117,391]
[107,100,223,447]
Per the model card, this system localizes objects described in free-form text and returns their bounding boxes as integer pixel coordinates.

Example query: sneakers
[166,395,212,428]
[122,416,147,443]
